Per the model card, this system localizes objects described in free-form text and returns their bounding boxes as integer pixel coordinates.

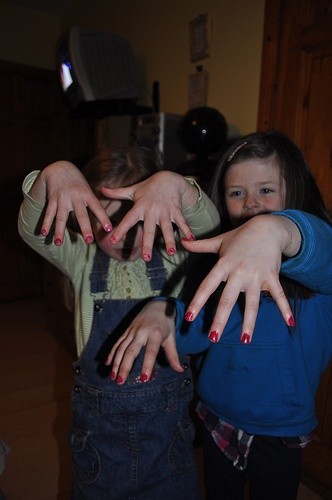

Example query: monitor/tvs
[58,26,145,113]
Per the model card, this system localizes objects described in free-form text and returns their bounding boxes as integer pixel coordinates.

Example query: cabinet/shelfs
[0,59,90,303]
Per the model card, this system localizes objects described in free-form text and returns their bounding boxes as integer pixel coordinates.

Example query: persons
[105,130,332,500]
[17,144,221,500]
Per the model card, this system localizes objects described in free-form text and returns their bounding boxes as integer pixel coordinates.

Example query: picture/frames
[189,13,213,63]
[188,72,207,109]
